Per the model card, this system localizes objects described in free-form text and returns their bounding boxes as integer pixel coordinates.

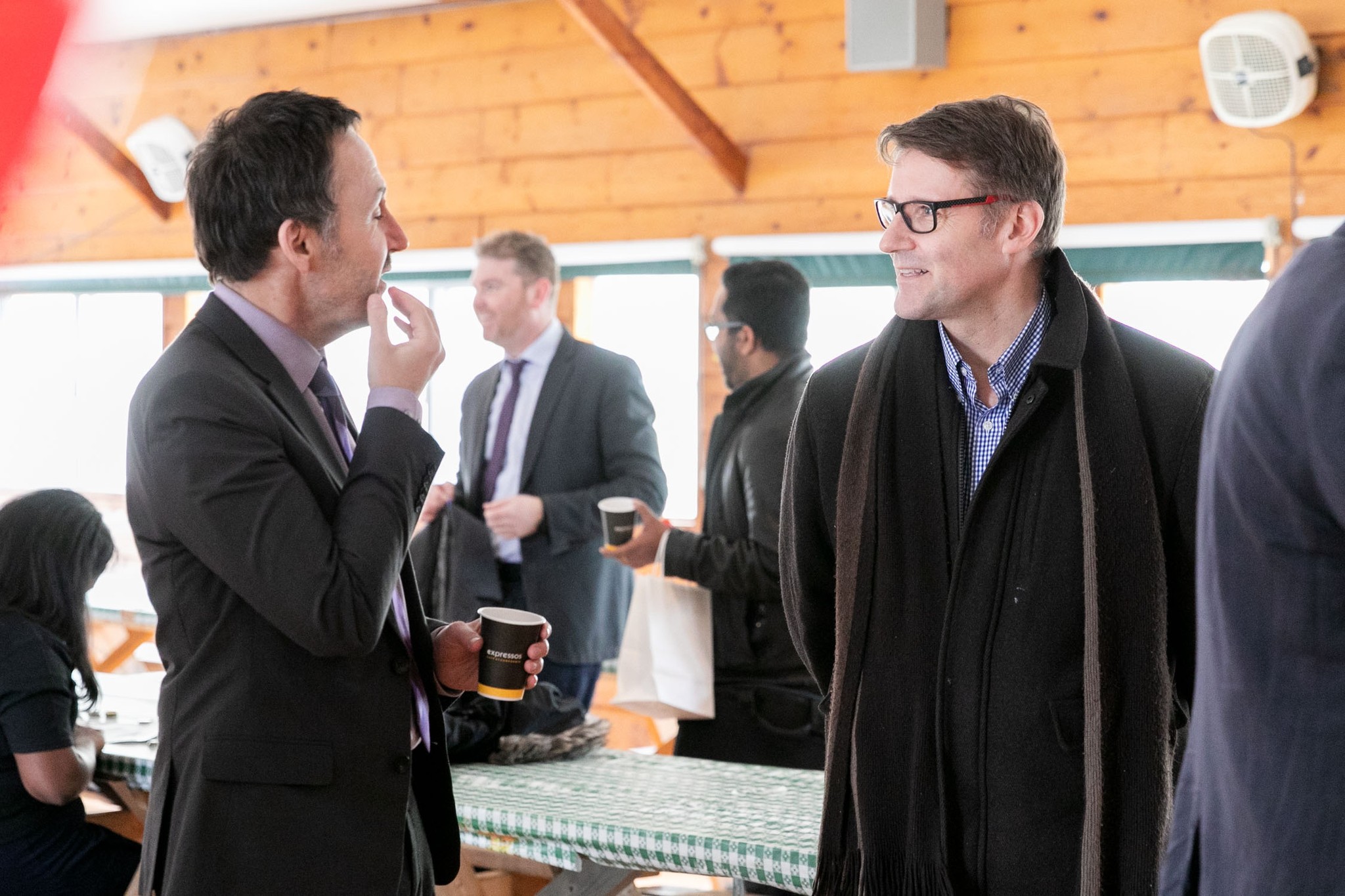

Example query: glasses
[702,316,749,340]
[874,196,1021,234]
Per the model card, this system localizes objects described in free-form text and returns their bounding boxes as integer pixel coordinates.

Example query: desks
[70,685,825,896]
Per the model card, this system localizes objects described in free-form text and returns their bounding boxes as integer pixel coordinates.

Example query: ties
[308,362,431,750]
[479,353,526,498]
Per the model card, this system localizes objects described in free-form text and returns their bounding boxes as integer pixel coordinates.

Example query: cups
[477,607,546,702]
[599,496,637,546]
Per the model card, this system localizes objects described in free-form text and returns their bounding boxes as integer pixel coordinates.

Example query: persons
[124,91,552,896]
[0,489,143,896]
[420,230,669,713]
[599,261,828,769]
[778,95,1221,896]
[1160,221,1345,895]
